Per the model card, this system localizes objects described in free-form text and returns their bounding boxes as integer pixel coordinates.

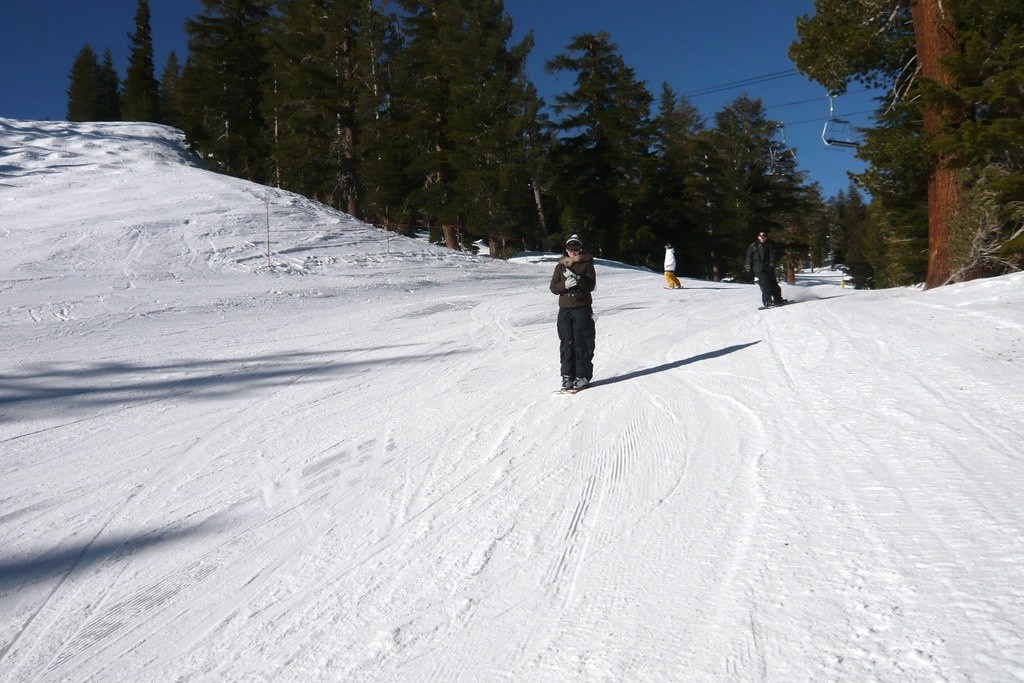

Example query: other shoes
[675,285,681,289]
[774,298,788,304]
[666,285,673,289]
[764,300,775,306]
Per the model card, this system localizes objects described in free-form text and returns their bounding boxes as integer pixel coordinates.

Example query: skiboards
[552,383,593,394]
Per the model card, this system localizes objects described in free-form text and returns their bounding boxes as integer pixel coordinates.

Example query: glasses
[566,246,579,251]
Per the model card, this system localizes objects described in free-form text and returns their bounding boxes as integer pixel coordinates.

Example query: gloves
[563,267,580,281]
[747,269,751,272]
[564,276,576,289]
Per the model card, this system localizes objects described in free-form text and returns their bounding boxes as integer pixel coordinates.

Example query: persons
[746,232,789,308]
[664,243,681,289]
[550,234,596,390]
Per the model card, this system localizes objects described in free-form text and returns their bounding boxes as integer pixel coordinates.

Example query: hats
[566,234,582,252]
[759,232,766,236]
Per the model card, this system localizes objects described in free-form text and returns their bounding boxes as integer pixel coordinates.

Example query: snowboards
[757,299,795,310]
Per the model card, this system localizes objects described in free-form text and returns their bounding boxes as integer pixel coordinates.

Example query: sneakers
[573,377,585,385]
[561,374,573,388]
